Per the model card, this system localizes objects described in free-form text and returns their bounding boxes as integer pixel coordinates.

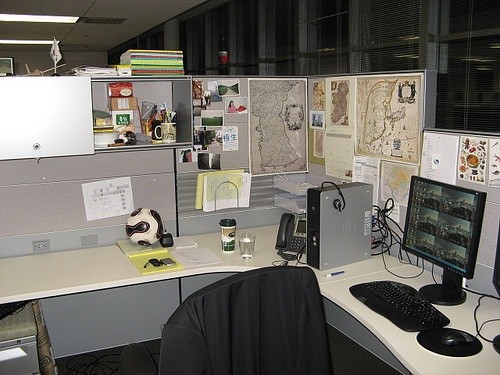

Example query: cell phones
[149,259,163,267]
[161,258,176,267]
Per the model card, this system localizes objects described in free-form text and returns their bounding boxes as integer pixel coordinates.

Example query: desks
[0,222,500,375]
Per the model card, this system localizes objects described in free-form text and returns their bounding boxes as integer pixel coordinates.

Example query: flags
[49,39,62,62]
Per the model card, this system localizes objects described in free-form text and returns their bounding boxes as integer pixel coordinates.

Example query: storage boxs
[95,81,138,131]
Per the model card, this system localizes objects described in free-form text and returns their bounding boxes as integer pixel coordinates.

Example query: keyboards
[349,281,451,332]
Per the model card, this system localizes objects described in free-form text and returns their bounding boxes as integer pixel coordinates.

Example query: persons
[228,101,236,113]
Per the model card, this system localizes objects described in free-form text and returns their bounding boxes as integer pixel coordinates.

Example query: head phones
[321,182,346,212]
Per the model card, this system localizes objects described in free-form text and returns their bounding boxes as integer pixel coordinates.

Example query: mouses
[441,330,473,346]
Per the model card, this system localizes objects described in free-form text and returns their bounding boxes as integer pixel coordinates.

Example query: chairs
[120,265,330,375]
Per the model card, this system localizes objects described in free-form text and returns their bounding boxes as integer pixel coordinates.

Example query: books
[121,49,185,76]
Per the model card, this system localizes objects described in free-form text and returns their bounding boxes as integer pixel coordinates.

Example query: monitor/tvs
[401,175,487,306]
[492,221,500,354]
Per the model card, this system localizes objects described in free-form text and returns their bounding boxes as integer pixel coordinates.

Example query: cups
[238,232,256,260]
[141,119,152,136]
[219,219,236,254]
[141,101,155,119]
[218,51,228,64]
[152,121,163,140]
[155,123,177,143]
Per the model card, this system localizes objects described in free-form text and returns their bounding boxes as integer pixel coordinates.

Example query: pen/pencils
[323,271,344,277]
[141,102,176,123]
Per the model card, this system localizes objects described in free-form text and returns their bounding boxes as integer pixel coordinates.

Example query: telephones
[275,213,307,254]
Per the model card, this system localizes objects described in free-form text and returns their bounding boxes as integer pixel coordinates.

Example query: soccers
[126,207,164,247]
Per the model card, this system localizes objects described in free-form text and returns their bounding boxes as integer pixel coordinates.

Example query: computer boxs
[306,181,374,270]
